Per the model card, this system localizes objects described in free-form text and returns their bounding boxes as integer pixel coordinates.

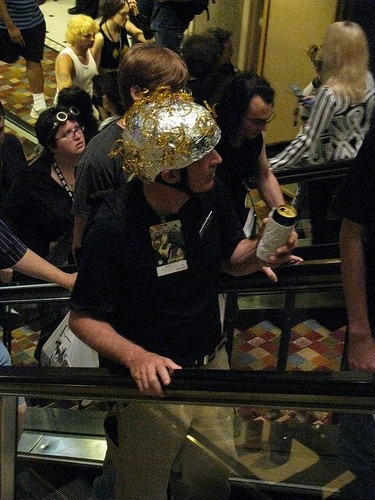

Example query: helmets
[120,88,220,187]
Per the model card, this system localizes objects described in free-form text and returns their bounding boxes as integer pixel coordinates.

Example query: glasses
[118,11,131,17]
[56,124,85,140]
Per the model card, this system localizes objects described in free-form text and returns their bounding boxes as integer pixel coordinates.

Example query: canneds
[272,205,298,226]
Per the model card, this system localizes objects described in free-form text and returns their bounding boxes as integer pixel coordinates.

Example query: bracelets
[5,20,14,28]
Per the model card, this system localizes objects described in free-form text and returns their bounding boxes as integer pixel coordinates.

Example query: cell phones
[290,82,314,106]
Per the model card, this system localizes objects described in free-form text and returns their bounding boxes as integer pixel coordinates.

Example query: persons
[70,88,298,500]
[1,102,28,219]
[0,217,78,451]
[269,21,375,244]
[69,0,239,126]
[71,36,190,277]
[209,69,304,282]
[11,105,86,360]
[0,0,57,111]
[338,109,375,479]
[290,43,325,135]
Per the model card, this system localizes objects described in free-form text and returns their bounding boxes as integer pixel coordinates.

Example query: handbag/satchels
[41,309,99,367]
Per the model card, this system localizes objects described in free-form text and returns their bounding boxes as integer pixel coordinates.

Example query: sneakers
[29,105,54,119]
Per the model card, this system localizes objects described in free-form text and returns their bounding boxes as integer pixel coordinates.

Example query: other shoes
[68,7,82,14]
